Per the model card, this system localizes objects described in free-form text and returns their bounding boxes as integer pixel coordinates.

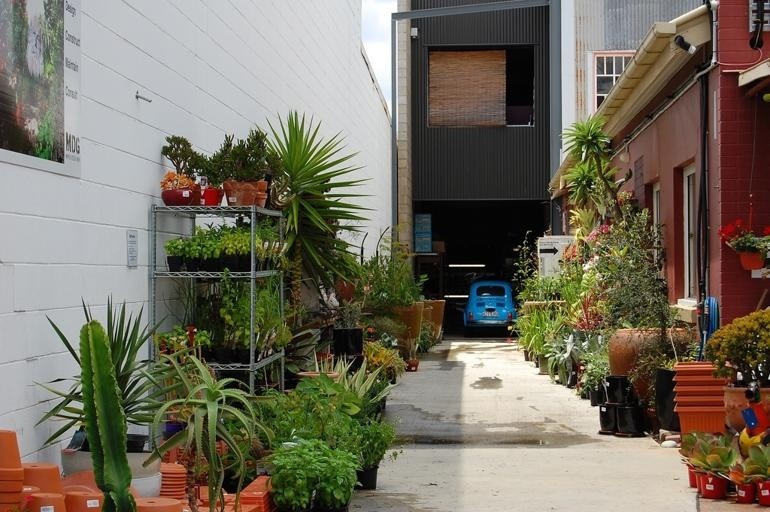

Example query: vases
[614,404,646,438]
[672,360,730,437]
[598,404,618,434]
[738,252,765,272]
[605,374,629,404]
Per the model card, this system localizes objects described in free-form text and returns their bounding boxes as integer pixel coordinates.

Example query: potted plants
[256,134,276,201]
[200,131,224,206]
[416,263,446,347]
[678,429,727,501]
[728,441,770,510]
[160,134,201,205]
[329,241,366,302]
[325,416,406,491]
[331,298,368,370]
[512,131,607,400]
[352,343,407,416]
[370,221,423,355]
[704,305,770,433]
[608,275,697,415]
[168,219,286,271]
[154,367,356,511]
[157,267,293,362]
[225,129,270,205]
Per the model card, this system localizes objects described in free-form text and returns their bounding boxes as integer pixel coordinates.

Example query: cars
[463,281,517,337]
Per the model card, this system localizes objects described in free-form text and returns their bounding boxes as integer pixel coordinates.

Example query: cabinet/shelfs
[150,204,286,397]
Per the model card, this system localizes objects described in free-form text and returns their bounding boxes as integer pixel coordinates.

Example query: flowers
[718,219,770,258]
